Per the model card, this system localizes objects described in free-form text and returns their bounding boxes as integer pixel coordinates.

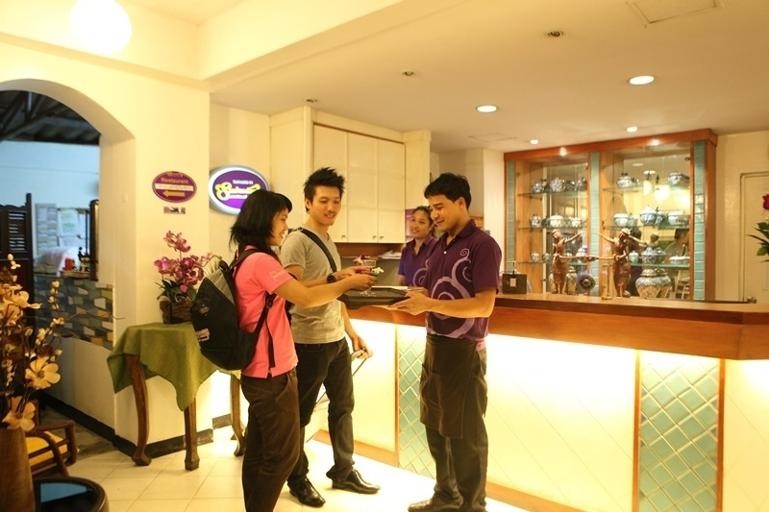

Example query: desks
[105,322,248,472]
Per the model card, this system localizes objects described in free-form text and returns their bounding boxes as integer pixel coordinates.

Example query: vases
[158,294,200,325]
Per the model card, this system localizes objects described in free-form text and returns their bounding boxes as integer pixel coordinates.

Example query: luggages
[304,348,373,442]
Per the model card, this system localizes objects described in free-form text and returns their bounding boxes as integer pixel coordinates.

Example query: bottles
[65,257,90,273]
[530,170,690,298]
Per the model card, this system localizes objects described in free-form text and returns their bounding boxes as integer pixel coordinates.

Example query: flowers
[742,192,769,267]
[153,226,223,303]
[0,251,88,434]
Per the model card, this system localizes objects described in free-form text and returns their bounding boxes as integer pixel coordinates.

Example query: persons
[226,189,378,512]
[386,172,502,511]
[630,232,660,250]
[286,166,380,508]
[598,227,631,296]
[663,229,690,290]
[550,229,583,294]
[397,206,438,288]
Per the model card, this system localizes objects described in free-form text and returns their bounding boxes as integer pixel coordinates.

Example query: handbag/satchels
[191,248,281,369]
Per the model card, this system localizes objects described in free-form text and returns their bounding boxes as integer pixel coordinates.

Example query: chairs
[23,398,81,480]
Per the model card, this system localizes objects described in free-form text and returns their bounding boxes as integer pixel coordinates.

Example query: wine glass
[359,259,384,295]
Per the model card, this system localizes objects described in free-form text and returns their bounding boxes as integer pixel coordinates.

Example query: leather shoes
[288,475,326,507]
[332,469,379,493]
[409,498,463,511]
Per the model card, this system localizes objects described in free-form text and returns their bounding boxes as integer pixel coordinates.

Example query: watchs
[327,273,336,284]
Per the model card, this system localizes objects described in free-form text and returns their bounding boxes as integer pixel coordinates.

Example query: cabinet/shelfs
[270,101,348,246]
[349,116,406,246]
[502,124,719,301]
